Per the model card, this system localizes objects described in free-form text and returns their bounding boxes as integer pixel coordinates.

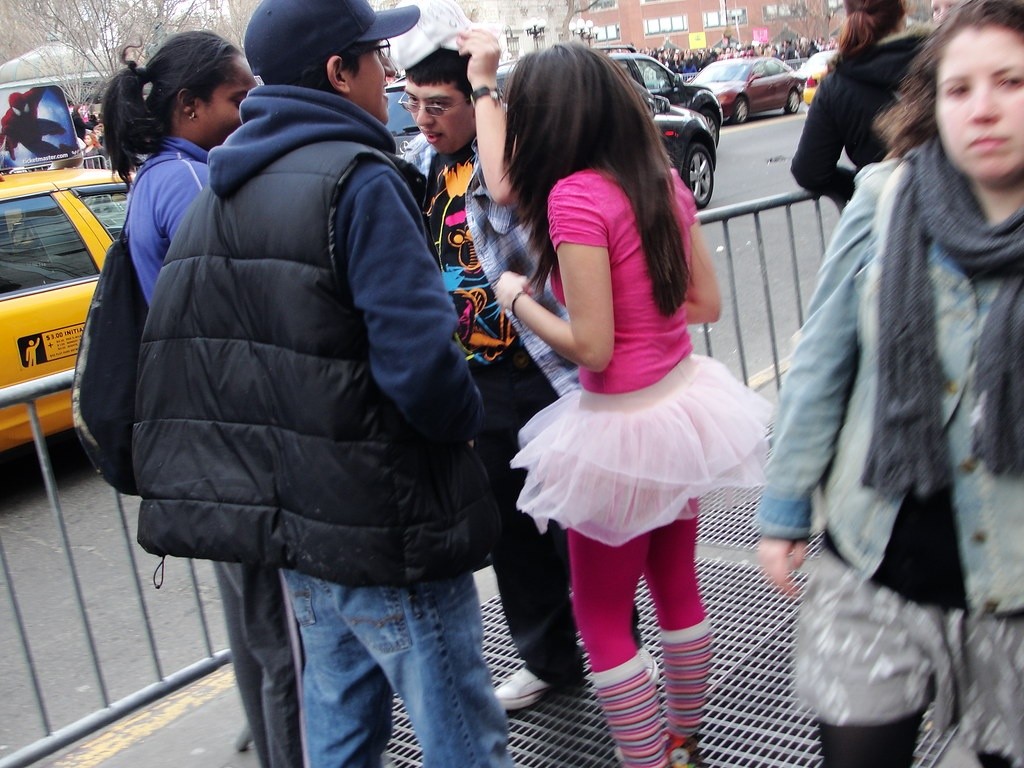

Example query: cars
[686,56,805,125]
[795,50,838,107]
[384,43,726,208]
[1,85,138,453]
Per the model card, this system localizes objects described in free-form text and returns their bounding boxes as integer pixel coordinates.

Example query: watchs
[471,85,504,108]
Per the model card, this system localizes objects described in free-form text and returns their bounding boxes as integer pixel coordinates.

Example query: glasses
[338,37,391,65]
[397,92,468,116]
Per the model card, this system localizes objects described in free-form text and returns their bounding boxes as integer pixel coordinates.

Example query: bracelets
[511,291,531,319]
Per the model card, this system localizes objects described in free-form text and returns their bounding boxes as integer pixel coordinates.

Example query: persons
[790,0,931,205]
[757,0,1024,767]
[601,36,839,73]
[71,104,110,169]
[496,43,772,768]
[104,31,303,767]
[397,27,660,711]
[136,0,517,768]
[931,0,960,20]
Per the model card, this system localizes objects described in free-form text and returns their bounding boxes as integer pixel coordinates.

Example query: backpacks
[70,153,191,498]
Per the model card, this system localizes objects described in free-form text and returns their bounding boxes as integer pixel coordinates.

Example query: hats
[244,0,421,77]
[383,0,483,71]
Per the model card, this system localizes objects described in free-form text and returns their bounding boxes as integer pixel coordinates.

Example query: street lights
[569,18,601,46]
[524,16,549,51]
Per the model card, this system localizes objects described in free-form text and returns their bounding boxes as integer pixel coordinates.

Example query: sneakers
[636,648,660,684]
[494,667,551,711]
